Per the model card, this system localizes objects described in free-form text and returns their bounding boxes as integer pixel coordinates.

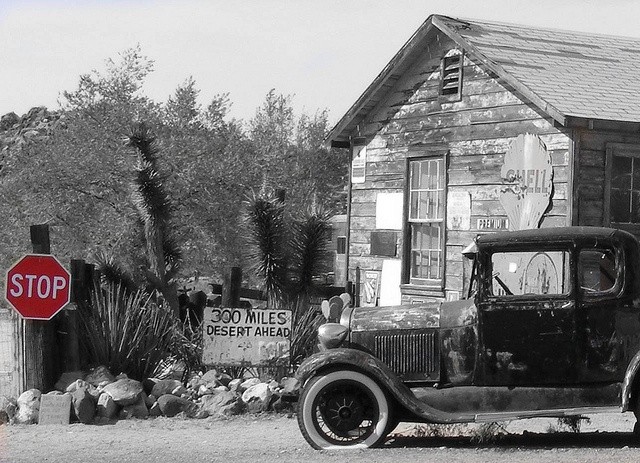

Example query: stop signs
[5,254,71,320]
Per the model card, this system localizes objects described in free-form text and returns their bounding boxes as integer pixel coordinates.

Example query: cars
[296,225,640,449]
